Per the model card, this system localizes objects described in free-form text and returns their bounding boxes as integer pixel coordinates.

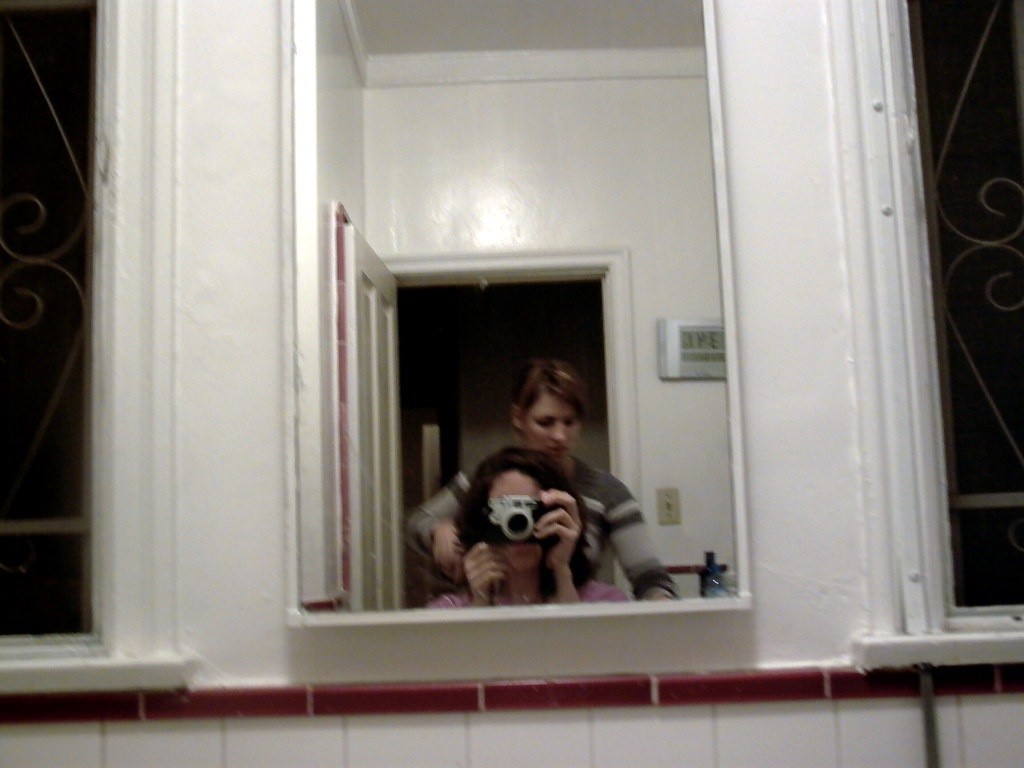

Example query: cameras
[482,494,560,545]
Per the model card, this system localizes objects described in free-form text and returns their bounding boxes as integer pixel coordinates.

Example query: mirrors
[273,0,750,625]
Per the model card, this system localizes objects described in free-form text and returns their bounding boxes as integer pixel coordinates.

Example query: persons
[408,360,680,600]
[425,446,630,609]
